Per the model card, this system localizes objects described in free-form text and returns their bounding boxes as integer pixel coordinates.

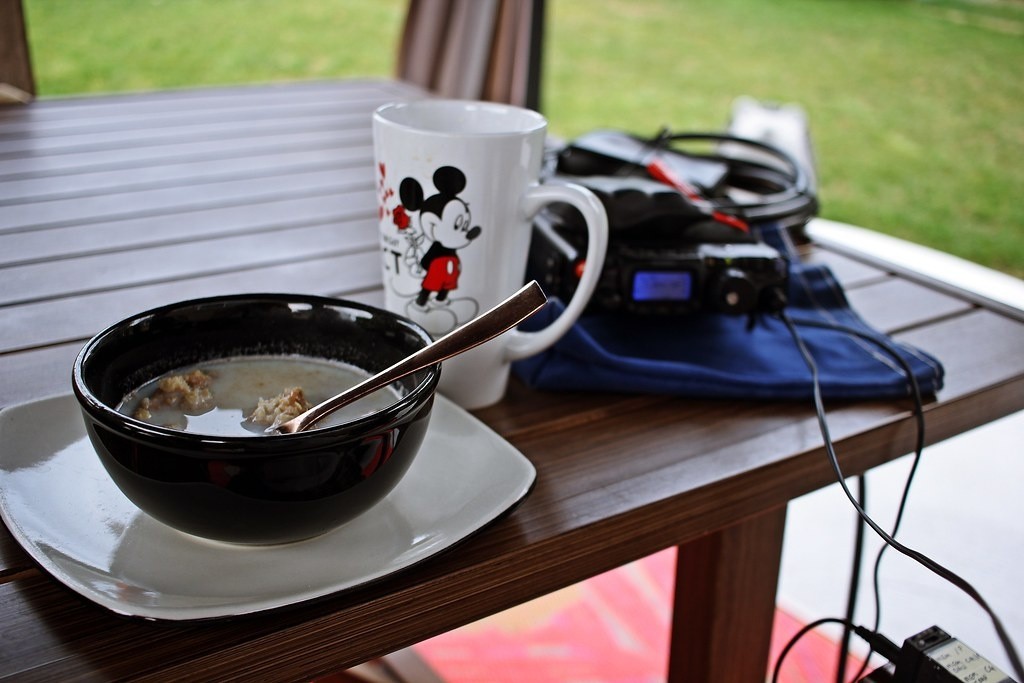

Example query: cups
[373,99,608,410]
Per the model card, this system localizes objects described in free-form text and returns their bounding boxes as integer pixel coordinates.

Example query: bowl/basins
[72,293,442,547]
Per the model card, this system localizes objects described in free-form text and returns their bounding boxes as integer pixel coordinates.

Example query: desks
[0,85,1022,683]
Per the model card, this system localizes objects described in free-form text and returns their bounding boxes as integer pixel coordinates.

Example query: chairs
[394,0,549,111]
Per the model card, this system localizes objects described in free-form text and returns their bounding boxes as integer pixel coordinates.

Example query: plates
[0,391,540,622]
